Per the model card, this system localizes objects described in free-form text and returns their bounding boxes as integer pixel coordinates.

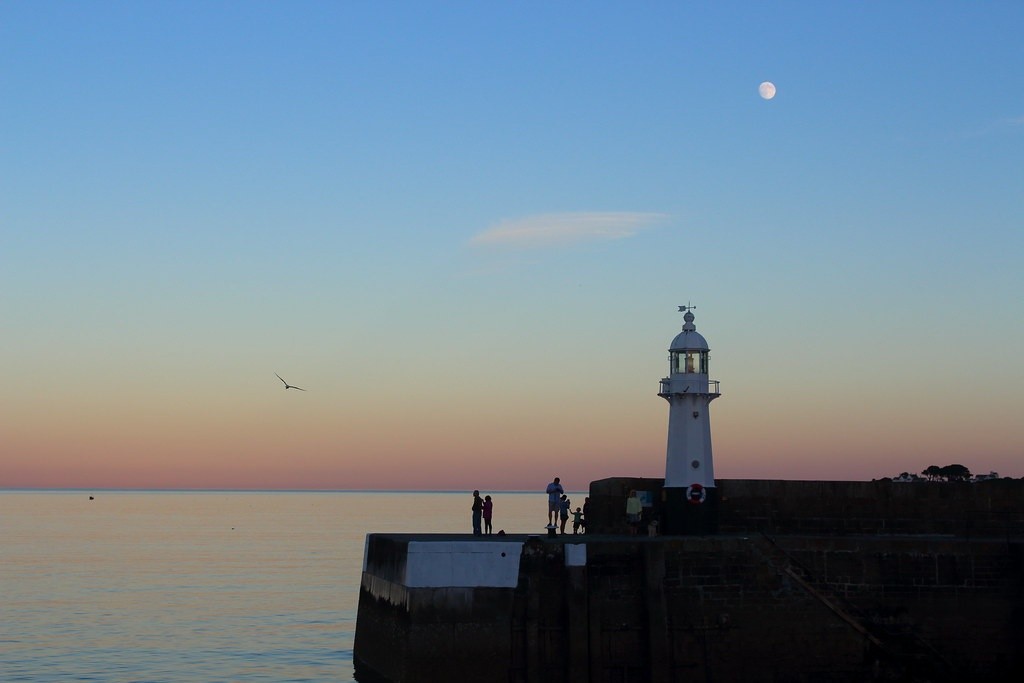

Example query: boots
[634,527,637,536]
[630,527,633,536]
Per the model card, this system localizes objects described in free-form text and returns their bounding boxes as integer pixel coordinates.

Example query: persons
[545,477,592,535]
[626,489,642,537]
[471,490,493,534]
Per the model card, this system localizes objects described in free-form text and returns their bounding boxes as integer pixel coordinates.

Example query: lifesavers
[685,483,707,506]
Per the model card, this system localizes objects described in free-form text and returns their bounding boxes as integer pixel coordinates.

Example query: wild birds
[273,373,306,392]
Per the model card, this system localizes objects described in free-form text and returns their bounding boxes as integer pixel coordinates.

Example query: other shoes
[554,524,557,526]
[548,523,552,526]
